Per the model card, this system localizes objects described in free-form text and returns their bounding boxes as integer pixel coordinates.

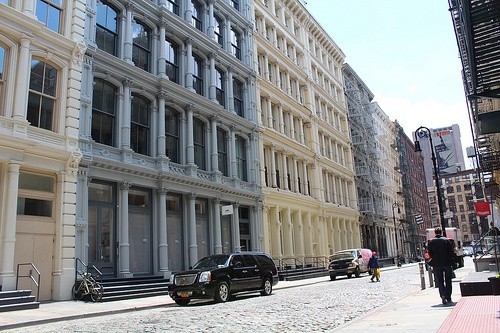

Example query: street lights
[391,202,402,255]
[413,126,447,237]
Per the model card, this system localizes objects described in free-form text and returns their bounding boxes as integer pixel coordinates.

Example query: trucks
[424,228,464,270]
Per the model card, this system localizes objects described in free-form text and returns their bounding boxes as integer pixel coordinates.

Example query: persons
[367,251,380,282]
[488,222,500,236]
[427,226,456,305]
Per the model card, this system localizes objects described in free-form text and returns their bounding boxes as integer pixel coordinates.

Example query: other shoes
[447,298,451,302]
[442,296,446,305]
[370,278,374,282]
[377,280,380,282]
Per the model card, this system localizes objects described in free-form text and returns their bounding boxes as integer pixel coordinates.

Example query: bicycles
[71,272,105,302]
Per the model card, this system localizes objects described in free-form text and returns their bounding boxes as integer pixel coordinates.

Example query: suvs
[168,252,279,305]
[329,248,372,279]
